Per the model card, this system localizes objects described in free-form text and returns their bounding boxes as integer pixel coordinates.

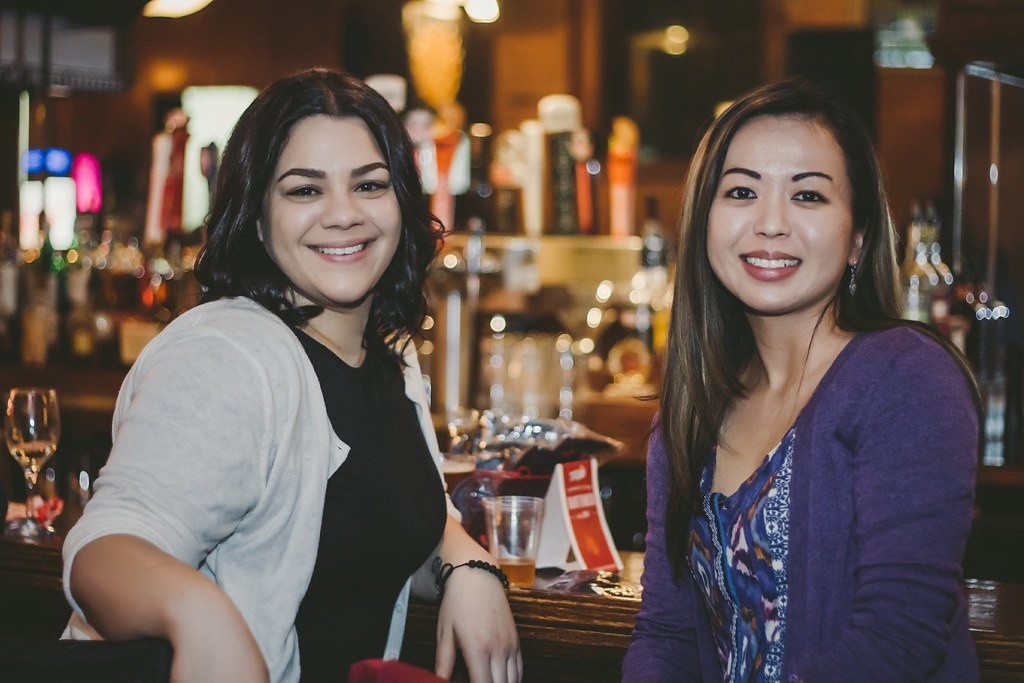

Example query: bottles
[633,194,671,400]
[893,198,1006,468]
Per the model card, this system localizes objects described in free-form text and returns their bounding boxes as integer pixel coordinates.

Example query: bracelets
[429,554,512,596]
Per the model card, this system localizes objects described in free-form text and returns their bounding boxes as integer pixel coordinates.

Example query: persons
[53,67,525,683]
[618,77,985,683]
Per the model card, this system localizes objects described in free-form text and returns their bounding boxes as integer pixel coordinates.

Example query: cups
[481,495,546,590]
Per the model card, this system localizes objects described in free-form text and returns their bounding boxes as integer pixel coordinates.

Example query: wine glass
[4,387,59,538]
